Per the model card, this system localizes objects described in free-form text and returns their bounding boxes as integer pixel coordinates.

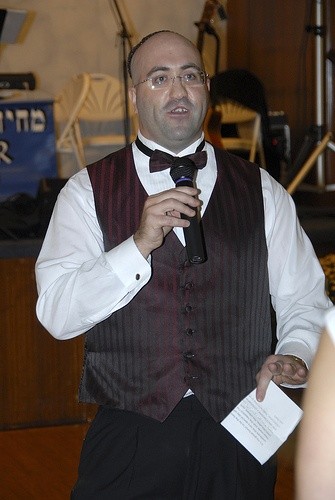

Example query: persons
[35,30,335,500]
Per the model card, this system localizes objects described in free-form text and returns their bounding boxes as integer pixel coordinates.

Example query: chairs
[54,72,140,170]
[210,69,268,170]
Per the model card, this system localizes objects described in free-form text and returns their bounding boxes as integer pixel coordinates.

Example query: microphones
[169,156,205,264]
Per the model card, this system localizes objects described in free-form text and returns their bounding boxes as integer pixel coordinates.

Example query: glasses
[133,71,209,90]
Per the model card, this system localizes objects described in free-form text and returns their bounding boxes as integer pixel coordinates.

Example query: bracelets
[290,354,306,366]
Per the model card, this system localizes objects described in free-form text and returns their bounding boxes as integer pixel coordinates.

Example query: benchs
[0,258,294,500]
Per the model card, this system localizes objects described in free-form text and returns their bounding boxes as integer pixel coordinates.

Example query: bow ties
[135,135,208,173]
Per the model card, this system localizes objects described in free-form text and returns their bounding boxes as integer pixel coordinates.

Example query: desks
[0,89,61,202]
